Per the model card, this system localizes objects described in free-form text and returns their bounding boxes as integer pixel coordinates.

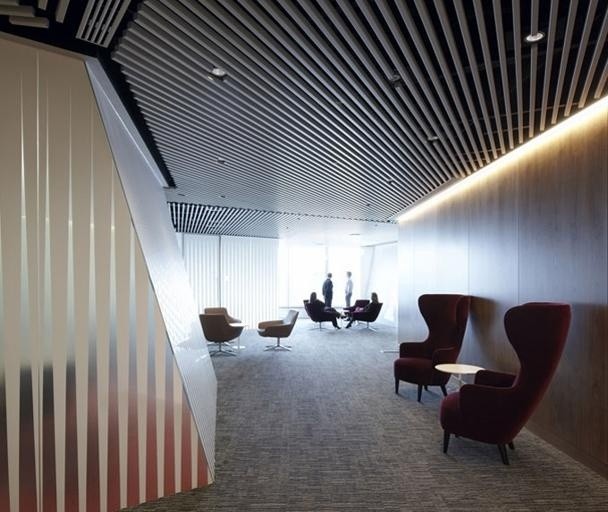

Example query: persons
[323,272,333,305]
[343,292,379,329]
[309,292,348,329]
[343,271,353,308]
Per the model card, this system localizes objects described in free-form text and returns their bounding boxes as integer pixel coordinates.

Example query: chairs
[198,300,383,358]
[392,295,571,466]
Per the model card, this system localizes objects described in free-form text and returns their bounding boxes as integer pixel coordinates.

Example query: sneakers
[334,315,351,329]
